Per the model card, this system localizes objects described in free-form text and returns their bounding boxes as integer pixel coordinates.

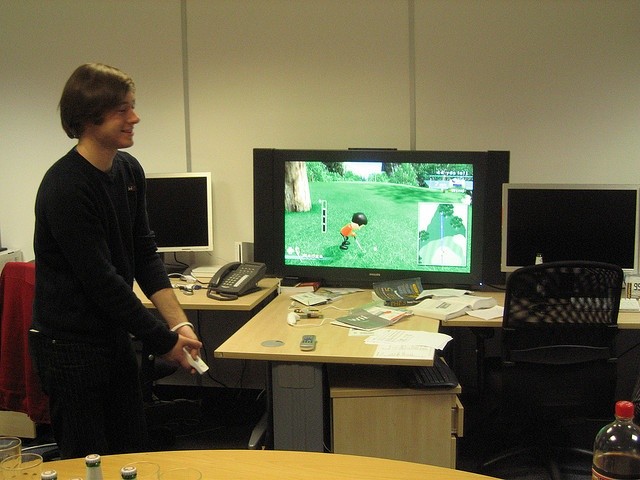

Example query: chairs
[477,260,623,479]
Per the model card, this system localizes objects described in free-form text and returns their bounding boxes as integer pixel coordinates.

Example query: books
[569,296,640,314]
[276,274,325,293]
[371,277,431,308]
[405,295,498,323]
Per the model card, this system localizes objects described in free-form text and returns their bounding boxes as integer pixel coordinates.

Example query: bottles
[591,401,640,480]
[85,454,103,480]
[41,470,57,480]
[120,466,137,480]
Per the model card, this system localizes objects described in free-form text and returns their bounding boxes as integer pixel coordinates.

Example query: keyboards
[408,352,459,389]
[618,298,640,314]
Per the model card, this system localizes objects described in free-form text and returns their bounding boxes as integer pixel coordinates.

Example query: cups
[0,437,22,480]
[623,274,640,298]
[0,453,43,480]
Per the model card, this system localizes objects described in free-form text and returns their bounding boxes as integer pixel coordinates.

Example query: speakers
[252,148,278,277]
[482,150,510,291]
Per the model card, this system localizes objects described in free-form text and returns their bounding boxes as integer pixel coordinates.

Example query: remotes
[299,334,316,351]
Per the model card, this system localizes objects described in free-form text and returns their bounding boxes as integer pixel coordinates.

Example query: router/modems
[191,266,223,278]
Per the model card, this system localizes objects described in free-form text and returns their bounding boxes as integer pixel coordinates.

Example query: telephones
[206,262,267,301]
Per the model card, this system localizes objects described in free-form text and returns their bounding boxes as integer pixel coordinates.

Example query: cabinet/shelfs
[329,357,466,469]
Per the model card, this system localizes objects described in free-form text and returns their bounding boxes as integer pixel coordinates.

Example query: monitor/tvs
[500,183,639,276]
[144,171,214,278]
[274,148,487,290]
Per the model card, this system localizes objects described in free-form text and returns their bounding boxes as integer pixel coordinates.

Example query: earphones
[288,301,295,309]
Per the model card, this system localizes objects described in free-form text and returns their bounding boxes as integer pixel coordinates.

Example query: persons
[29,64,206,456]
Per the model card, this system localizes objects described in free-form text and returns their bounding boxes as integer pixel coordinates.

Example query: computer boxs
[272,360,324,453]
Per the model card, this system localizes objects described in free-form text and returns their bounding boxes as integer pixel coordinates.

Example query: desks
[214,287,441,367]
[440,291,638,328]
[132,278,282,312]
[1,450,496,480]
